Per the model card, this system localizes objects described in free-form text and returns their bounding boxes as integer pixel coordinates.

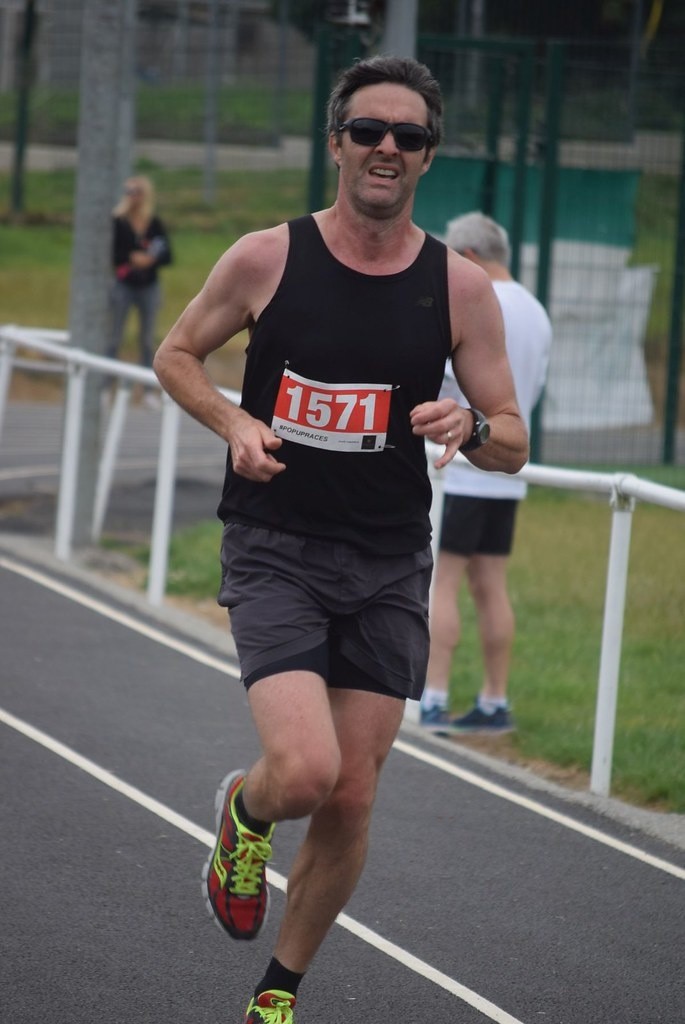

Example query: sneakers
[452,705,511,734]
[244,989,296,1024]
[200,768,276,942]
[421,707,454,735]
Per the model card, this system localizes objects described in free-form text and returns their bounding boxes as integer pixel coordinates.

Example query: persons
[109,55,528,1024]
[419,215,553,740]
[105,175,175,412]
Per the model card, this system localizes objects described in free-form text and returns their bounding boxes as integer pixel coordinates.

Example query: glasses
[338,116,433,152]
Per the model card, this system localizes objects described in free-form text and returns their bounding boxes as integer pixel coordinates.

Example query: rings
[446,430,452,443]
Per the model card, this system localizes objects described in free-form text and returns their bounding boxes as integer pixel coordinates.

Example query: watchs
[458,408,491,456]
